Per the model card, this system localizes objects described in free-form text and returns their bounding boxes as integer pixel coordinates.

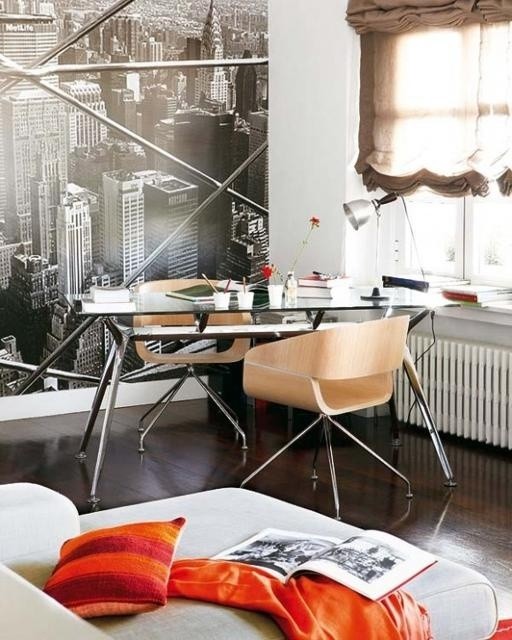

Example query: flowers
[261,216,320,288]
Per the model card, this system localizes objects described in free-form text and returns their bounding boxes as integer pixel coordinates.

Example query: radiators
[392,331,512,453]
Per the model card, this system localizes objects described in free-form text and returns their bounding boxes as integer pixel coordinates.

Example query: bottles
[285,274,297,306]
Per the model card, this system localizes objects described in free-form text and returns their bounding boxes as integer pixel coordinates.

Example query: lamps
[343,191,397,301]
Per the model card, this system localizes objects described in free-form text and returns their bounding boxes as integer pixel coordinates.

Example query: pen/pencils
[202,273,219,294]
[243,276,246,292]
[224,279,232,292]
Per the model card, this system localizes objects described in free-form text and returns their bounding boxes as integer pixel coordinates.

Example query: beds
[0,486,498,640]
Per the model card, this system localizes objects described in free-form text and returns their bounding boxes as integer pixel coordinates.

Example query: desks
[62,278,462,506]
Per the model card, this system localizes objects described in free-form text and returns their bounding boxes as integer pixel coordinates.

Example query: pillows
[0,481,185,619]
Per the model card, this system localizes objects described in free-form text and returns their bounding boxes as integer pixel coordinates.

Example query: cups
[237,292,254,310]
[213,292,231,311]
[268,285,283,307]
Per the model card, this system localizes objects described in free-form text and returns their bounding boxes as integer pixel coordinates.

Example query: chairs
[133,278,253,452]
[236,315,413,520]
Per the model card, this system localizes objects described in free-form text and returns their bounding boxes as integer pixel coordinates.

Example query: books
[298,274,352,289]
[382,274,471,288]
[81,286,137,314]
[297,286,355,299]
[442,298,512,309]
[167,277,269,302]
[209,526,440,604]
[441,286,512,303]
[383,283,470,293]
